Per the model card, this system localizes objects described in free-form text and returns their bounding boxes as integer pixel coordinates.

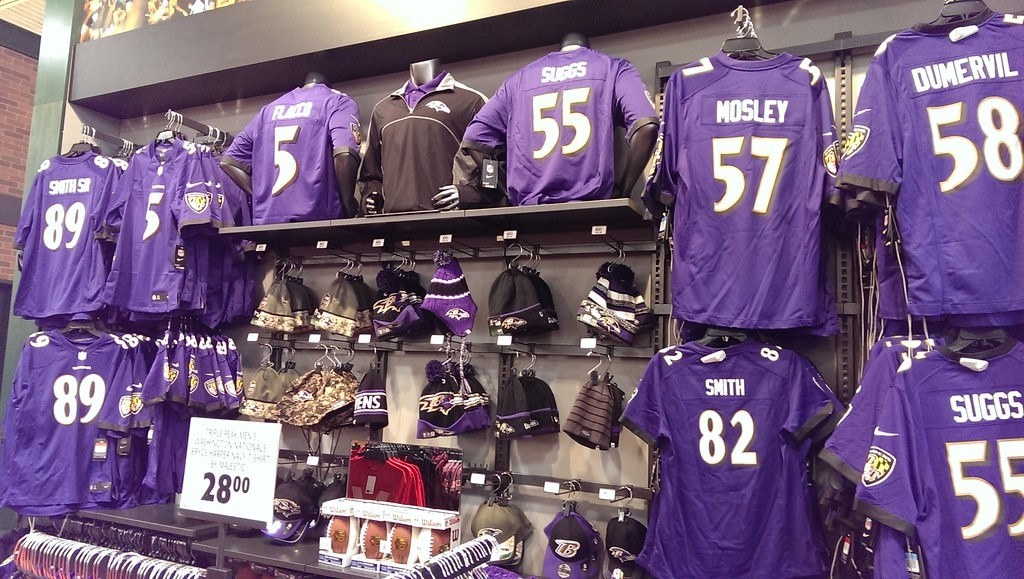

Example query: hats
[238,255,652,437]
[261,469,350,543]
[471,496,648,579]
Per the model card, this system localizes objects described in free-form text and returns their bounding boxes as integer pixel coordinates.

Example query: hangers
[384,527,504,579]
[644,0,1024,418]
[26,109,227,368]
[12,528,212,579]
